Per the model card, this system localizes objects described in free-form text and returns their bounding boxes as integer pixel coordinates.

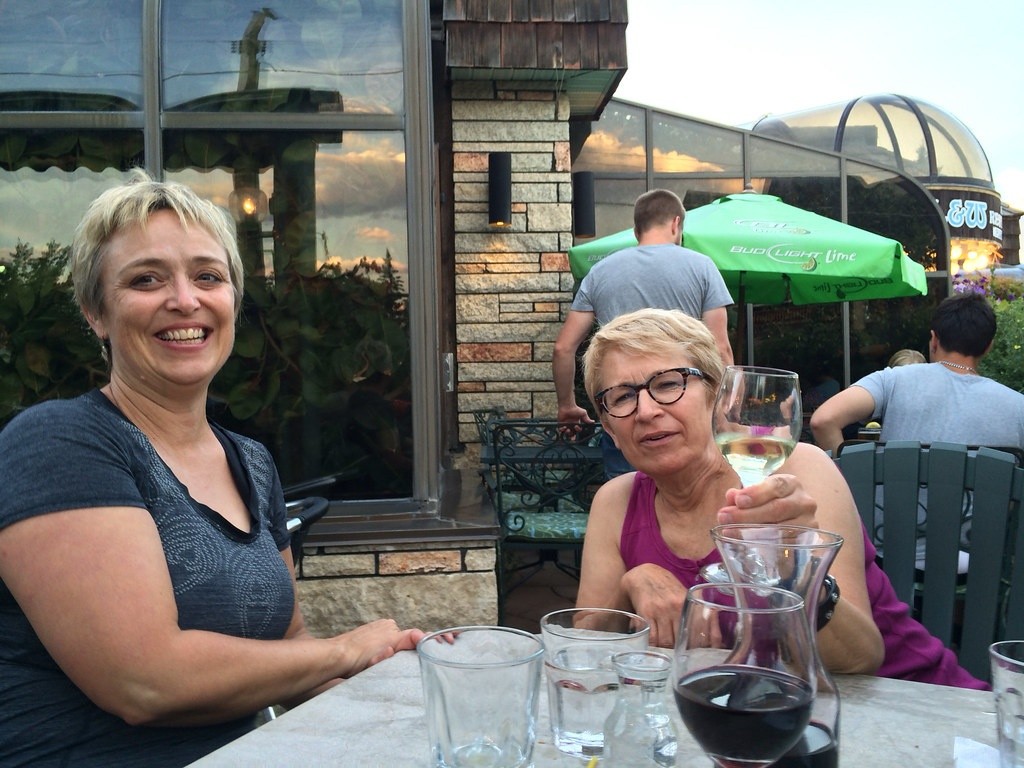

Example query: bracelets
[818,574,841,633]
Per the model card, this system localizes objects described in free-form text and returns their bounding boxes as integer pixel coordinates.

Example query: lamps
[573,171,595,238]
[229,187,274,240]
[488,151,512,228]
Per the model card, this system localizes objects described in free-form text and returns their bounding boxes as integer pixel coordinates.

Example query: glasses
[595,366,706,418]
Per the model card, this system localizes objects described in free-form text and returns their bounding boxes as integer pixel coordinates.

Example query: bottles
[710,523,845,768]
[601,650,677,768]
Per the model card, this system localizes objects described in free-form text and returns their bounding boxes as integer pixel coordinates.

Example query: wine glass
[700,366,804,598]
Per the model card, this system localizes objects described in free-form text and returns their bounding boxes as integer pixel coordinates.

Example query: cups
[417,625,546,767]
[988,640,1024,768]
[671,583,818,768]
[540,608,651,760]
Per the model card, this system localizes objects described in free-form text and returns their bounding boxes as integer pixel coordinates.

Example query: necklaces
[939,361,978,374]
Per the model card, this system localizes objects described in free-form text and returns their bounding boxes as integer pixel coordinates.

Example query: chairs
[825,440,1023,684]
[472,405,615,626]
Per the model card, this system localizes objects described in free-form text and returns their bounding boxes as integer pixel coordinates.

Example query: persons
[551,190,734,436]
[888,349,927,368]
[572,307,992,691]
[0,164,459,768]
[811,290,1024,588]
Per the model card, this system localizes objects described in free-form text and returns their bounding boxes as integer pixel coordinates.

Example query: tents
[566,183,927,371]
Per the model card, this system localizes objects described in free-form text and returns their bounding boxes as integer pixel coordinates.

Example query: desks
[479,446,604,462]
[182,627,998,768]
